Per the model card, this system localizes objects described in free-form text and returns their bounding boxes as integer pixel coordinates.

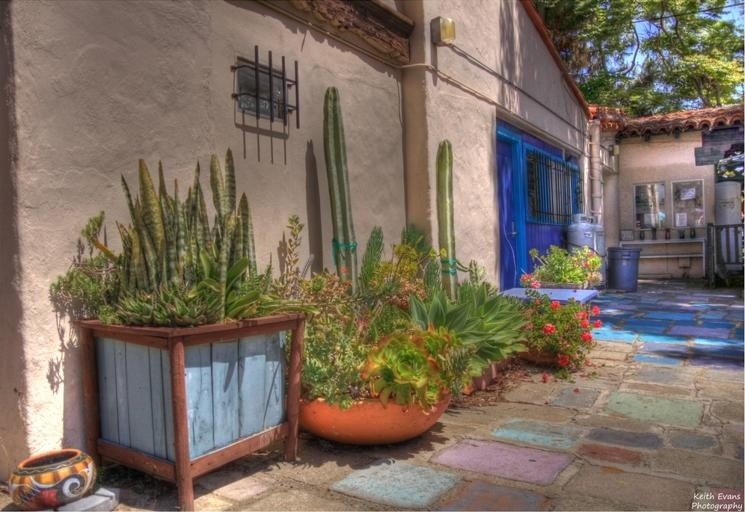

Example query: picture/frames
[671,178,705,228]
[633,180,666,231]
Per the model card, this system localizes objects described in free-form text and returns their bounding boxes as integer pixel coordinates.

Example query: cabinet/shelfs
[619,237,708,276]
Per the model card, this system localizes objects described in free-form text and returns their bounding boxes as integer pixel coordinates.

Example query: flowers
[517,292,603,367]
[520,243,604,290]
[270,210,484,403]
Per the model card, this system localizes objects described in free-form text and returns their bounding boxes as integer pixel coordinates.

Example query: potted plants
[407,280,530,389]
[48,146,311,512]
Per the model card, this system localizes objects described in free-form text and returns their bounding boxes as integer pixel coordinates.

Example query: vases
[520,346,554,364]
[8,448,98,512]
[300,388,453,447]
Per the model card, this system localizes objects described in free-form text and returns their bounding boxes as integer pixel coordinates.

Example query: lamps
[430,16,456,46]
[609,144,619,156]
